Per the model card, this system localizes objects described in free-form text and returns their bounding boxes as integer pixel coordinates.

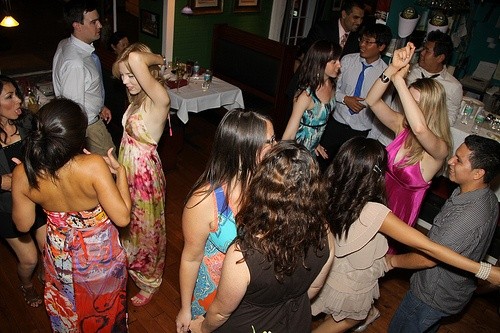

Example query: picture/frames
[188,0,224,15]
[231,0,262,13]
[140,9,160,38]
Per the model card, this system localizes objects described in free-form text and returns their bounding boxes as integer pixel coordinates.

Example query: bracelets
[379,74,392,86]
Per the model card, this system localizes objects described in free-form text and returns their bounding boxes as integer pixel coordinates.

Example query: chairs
[460,59,499,101]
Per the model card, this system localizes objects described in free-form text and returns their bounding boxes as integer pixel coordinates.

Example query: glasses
[265,135,276,148]
[358,36,377,46]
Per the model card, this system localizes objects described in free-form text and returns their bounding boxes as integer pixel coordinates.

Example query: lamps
[0,0,20,28]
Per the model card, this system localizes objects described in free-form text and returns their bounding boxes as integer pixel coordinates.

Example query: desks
[442,110,500,202]
[164,73,245,156]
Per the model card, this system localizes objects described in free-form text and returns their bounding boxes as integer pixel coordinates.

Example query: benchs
[197,24,297,139]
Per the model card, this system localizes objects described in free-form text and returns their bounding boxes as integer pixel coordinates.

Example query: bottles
[202,69,211,90]
[460,101,474,124]
[472,108,486,135]
[193,61,200,79]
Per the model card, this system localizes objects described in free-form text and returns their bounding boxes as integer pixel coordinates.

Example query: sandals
[131,289,160,307]
[17,280,43,307]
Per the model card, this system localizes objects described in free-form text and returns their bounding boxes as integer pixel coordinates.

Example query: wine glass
[175,67,185,94]
[186,61,193,81]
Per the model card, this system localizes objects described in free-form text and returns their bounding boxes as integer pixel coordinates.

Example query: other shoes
[352,305,381,332]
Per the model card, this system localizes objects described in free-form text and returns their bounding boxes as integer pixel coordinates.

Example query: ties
[341,34,348,45]
[419,72,440,79]
[349,62,373,115]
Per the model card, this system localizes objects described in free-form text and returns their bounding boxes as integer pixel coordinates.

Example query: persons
[310,137,500,333]
[189,140,334,333]
[102,32,130,131]
[384,135,500,333]
[51,0,119,181]
[11,95,133,333]
[174,111,278,333]
[111,42,172,307]
[280,0,462,173]
[0,76,48,308]
[365,42,454,255]
